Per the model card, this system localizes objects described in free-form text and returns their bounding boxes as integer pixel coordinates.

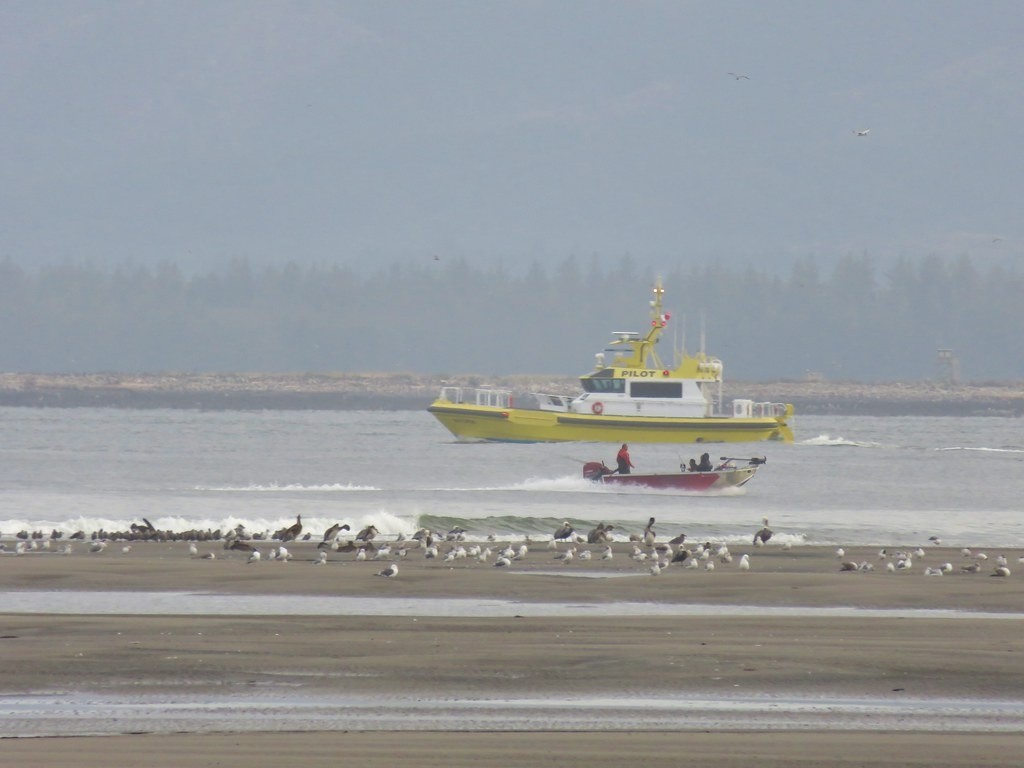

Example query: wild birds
[836,537,1024,578]
[1,515,750,580]
[728,72,750,80]
[753,516,772,547]
[850,129,870,136]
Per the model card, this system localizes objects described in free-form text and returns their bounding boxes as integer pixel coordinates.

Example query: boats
[582,458,766,491]
[426,273,795,445]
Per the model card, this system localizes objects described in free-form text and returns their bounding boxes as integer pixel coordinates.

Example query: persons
[617,444,634,474]
[688,453,711,472]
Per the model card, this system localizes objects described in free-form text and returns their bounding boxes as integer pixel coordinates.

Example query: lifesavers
[592,402,604,414]
